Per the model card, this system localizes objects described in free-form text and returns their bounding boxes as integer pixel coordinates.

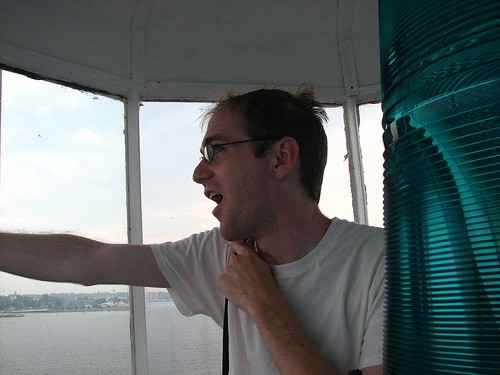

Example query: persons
[0,82,385,375]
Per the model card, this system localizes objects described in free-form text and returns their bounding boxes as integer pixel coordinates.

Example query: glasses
[201,137,270,165]
